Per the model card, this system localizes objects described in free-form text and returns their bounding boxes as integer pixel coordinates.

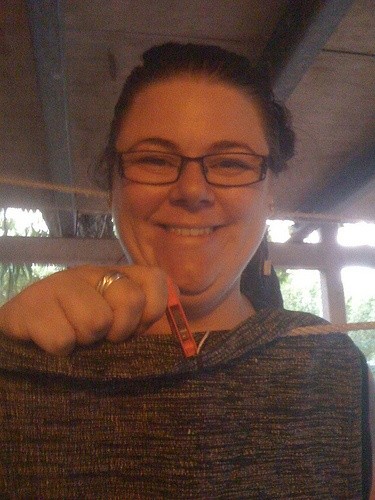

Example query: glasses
[109,148,275,191]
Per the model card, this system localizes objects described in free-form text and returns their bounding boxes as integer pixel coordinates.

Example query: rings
[96,270,128,297]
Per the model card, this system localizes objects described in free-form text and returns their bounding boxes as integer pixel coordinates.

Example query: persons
[0,42,372,500]
